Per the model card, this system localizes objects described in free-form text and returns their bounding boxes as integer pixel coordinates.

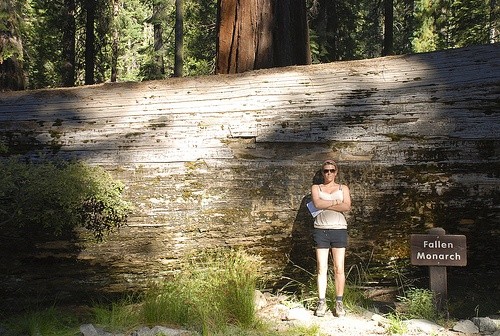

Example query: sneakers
[335,300,346,317]
[314,299,327,317]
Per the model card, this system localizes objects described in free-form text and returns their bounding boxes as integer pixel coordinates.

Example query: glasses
[322,169,336,173]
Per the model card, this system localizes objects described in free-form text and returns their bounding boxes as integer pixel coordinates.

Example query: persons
[311,160,351,317]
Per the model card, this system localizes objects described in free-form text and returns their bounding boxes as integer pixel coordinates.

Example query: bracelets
[332,200,339,206]
